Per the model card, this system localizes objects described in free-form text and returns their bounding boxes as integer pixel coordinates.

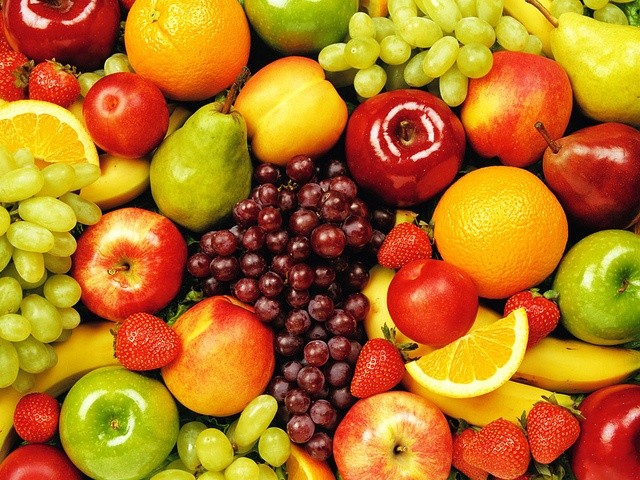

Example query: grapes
[188,156,396,464]
[151,394,290,480]
[318,0,637,108]
[0,145,103,391]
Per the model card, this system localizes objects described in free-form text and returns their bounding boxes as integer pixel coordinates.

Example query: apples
[346,88,465,205]
[1,444,81,480]
[159,294,275,416]
[553,229,640,346]
[244,0,359,52]
[73,208,187,321]
[4,0,120,70]
[460,50,573,165]
[60,366,179,480]
[332,391,453,480]
[573,383,640,479]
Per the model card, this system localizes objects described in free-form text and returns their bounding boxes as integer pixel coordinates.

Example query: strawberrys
[0,49,29,101]
[112,311,182,372]
[29,60,81,108]
[351,336,406,399]
[527,401,580,465]
[13,394,60,443]
[463,416,532,480]
[503,292,559,351]
[378,221,433,268]
[451,430,489,480]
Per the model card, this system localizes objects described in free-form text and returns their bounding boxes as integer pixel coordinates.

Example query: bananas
[361,267,640,427]
[0,320,120,463]
[501,0,557,59]
[77,103,189,208]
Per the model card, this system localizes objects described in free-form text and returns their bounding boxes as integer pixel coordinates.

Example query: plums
[82,72,170,158]
[235,56,348,164]
[387,259,478,347]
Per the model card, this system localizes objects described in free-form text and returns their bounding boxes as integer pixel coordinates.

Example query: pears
[150,67,254,230]
[528,0,640,126]
[534,121,640,227]
